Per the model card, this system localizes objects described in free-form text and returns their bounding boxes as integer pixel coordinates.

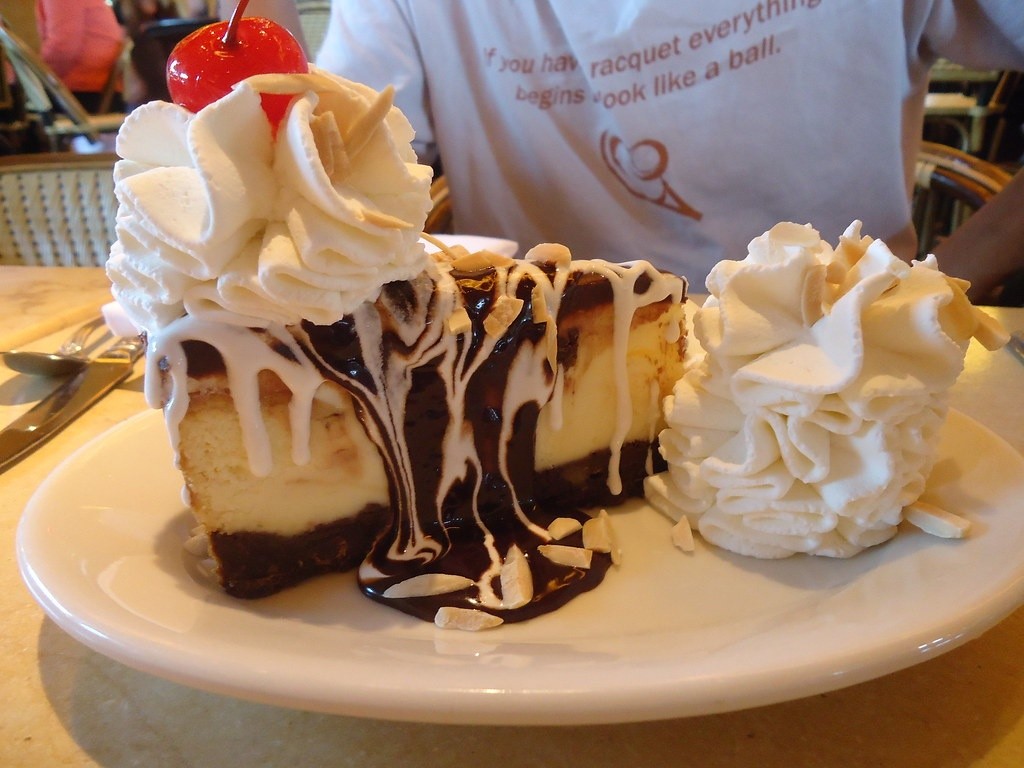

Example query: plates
[18,407,1023,727]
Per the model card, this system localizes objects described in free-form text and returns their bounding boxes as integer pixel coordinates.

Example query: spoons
[0,316,106,376]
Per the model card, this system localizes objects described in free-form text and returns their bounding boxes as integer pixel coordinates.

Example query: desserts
[104,0,1003,594]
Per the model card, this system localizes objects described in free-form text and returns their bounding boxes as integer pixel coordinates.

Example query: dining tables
[0,267,1024,767]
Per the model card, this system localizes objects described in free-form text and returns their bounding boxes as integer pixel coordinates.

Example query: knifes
[0,336,144,466]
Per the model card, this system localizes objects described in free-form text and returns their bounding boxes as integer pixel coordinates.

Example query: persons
[31,0,132,115]
[310,0,1024,310]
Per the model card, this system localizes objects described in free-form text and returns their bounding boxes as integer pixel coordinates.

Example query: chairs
[0,152,123,266]
[93,37,135,114]
[920,69,1022,164]
[134,17,222,106]
[0,19,131,153]
[420,138,1024,306]
